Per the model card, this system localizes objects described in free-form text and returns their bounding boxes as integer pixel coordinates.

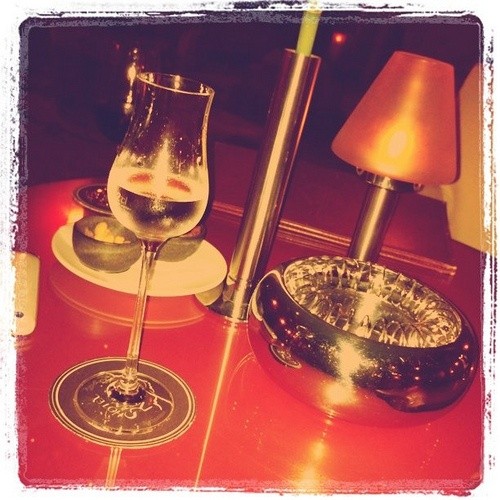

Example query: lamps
[328,51,459,266]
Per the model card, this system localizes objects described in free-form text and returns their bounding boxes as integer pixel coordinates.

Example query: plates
[49,224,227,298]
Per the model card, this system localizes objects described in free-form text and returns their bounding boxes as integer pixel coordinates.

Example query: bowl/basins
[72,215,143,275]
[154,221,207,262]
[71,182,113,217]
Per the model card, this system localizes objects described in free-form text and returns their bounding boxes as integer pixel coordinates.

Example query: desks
[18,174,480,486]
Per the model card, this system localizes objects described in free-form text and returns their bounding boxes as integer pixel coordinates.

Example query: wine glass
[48,70,214,447]
